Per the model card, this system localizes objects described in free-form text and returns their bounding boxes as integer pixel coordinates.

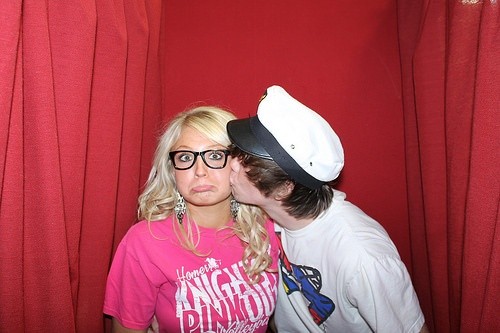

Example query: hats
[226,85,345,190]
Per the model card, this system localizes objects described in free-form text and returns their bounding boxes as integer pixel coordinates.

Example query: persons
[145,84,431,332]
[103,104,279,332]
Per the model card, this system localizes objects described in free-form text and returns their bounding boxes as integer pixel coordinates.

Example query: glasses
[169,149,231,170]
